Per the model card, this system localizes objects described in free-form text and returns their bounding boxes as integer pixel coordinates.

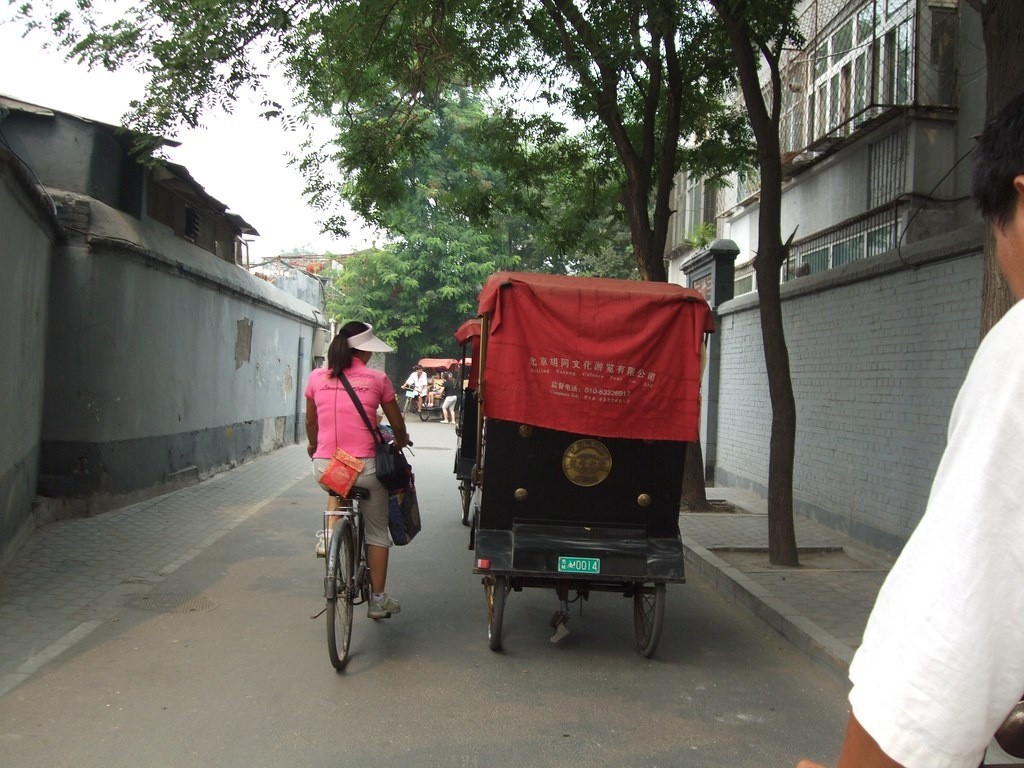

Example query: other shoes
[440,420,449,424]
[412,407,418,414]
[450,420,456,425]
[426,403,434,408]
[418,409,423,415]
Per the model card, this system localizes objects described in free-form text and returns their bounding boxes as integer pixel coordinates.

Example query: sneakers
[367,593,401,618]
[315,528,333,555]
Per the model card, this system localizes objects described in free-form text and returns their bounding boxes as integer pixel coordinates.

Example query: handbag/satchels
[375,442,407,490]
[388,442,421,546]
[317,446,366,499]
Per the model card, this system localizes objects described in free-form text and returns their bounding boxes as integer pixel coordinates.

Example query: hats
[346,322,394,353]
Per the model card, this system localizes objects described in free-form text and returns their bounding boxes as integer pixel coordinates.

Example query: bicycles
[310,440,415,669]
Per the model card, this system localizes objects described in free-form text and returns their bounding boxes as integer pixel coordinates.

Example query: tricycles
[402,357,458,422]
[453,272,714,657]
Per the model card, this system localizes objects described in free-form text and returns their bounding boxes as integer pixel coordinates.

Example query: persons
[304,321,409,619]
[794,91,1024,768]
[401,365,457,424]
